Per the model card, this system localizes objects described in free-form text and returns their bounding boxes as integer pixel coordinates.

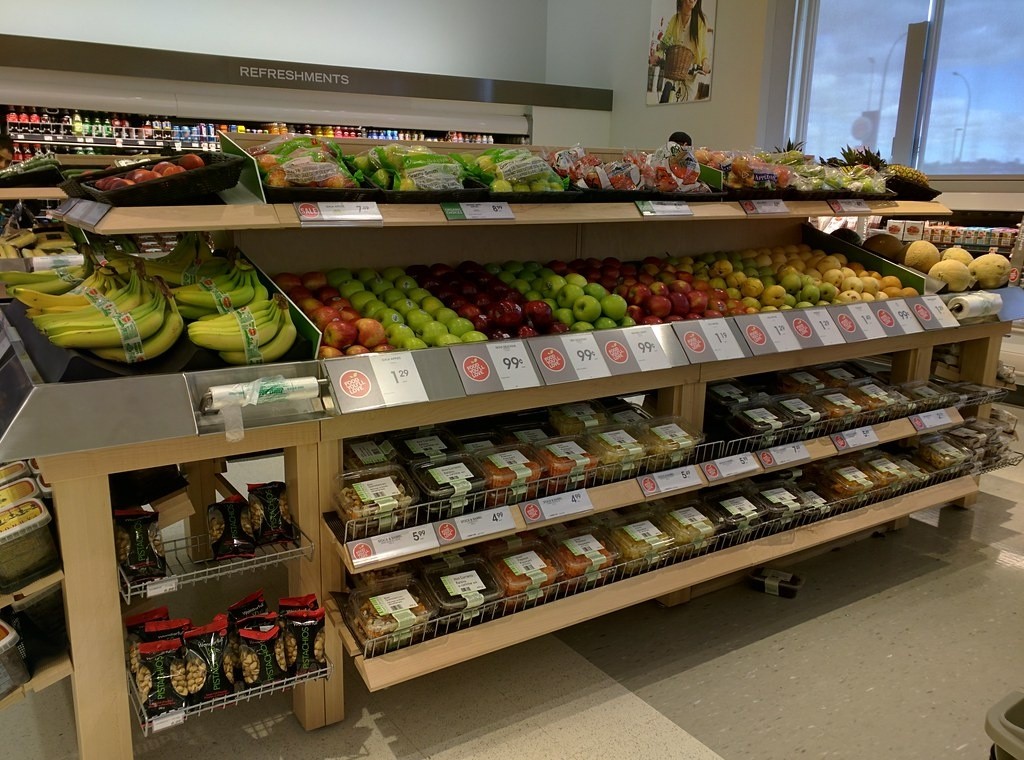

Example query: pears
[665,243,918,315]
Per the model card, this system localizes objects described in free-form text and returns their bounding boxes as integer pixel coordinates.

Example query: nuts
[108,486,327,718]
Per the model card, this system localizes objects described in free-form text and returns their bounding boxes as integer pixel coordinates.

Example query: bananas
[0,232,297,365]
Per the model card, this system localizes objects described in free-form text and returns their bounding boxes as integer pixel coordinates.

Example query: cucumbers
[0,150,104,180]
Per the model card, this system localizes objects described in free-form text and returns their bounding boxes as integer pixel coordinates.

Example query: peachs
[96,154,205,191]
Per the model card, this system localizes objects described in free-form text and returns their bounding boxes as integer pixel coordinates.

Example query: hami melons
[828,229,1012,293]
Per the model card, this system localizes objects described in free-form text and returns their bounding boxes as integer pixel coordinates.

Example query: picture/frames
[644,0,719,107]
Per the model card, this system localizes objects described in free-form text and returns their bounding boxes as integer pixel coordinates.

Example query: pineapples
[773,138,930,188]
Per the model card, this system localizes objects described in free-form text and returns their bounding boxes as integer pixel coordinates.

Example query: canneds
[172,122,269,142]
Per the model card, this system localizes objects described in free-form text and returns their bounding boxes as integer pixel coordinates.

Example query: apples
[693,145,791,191]
[250,133,565,197]
[275,250,754,359]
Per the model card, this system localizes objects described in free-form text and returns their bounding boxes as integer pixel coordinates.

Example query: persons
[669,131,691,147]
[649,0,710,102]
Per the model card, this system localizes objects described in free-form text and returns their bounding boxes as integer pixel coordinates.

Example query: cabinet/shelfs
[0,120,1023,760]
